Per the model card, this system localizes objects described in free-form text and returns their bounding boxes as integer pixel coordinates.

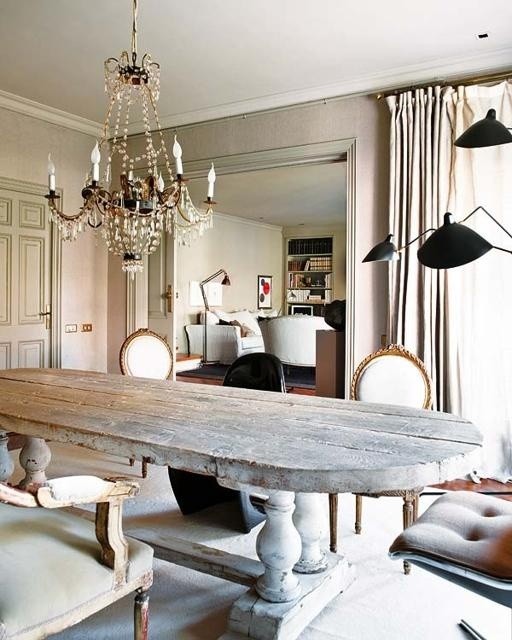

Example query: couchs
[184,308,280,362]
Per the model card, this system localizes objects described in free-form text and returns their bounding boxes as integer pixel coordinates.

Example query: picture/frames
[257,274,273,310]
[290,304,314,317]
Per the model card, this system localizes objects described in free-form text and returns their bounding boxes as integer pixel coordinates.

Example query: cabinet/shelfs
[283,232,337,317]
[314,328,344,399]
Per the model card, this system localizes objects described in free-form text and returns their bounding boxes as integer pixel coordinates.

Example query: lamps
[415,205,512,271]
[47,0,219,281]
[356,204,510,275]
[192,267,231,368]
[455,108,512,149]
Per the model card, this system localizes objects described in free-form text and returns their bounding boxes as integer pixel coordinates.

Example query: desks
[0,358,486,638]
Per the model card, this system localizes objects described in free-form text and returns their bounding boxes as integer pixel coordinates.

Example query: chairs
[321,338,436,577]
[118,326,177,480]
[1,422,157,639]
[255,313,336,377]
[164,348,292,535]
[387,485,512,609]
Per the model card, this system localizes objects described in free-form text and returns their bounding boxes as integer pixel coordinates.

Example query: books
[288,237,332,302]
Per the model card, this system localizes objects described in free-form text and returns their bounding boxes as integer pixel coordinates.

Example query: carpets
[176,361,320,391]
